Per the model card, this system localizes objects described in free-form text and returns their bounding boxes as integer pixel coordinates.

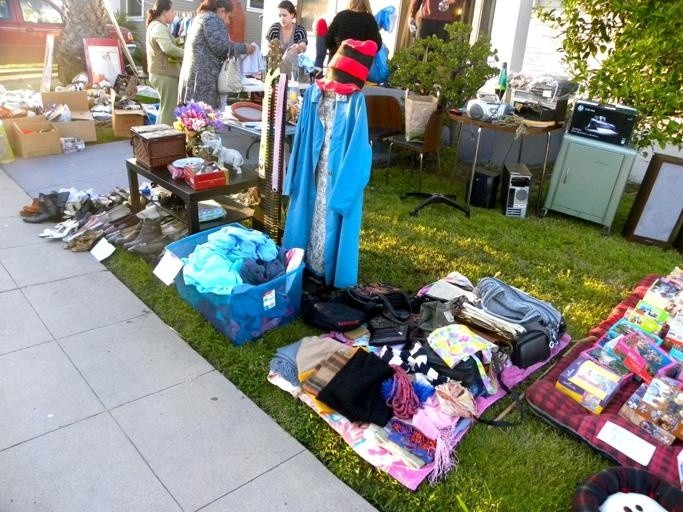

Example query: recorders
[467,91,515,120]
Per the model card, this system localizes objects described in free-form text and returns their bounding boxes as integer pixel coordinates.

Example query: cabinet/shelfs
[539,123,639,238]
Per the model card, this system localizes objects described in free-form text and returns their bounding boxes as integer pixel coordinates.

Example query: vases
[193,141,215,161]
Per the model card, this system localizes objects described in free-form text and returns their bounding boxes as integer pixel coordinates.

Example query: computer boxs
[502,160,534,217]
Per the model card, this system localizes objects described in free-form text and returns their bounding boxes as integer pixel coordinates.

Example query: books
[61,137,85,153]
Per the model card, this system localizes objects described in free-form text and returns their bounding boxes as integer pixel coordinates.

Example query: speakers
[467,169,501,209]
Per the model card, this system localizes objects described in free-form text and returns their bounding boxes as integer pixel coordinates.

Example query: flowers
[171,95,226,148]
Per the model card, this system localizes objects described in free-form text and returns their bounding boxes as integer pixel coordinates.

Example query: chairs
[361,93,407,188]
[379,99,449,196]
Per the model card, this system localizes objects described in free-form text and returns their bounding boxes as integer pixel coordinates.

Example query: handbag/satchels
[166,34,187,65]
[404,87,441,144]
[302,271,565,388]
[217,58,242,94]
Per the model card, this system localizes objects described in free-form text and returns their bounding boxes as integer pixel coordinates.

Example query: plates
[231,101,265,121]
[172,157,203,169]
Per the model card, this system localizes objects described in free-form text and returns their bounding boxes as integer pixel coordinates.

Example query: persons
[145,0,185,125]
[325,0,381,61]
[177,0,255,110]
[265,0,309,54]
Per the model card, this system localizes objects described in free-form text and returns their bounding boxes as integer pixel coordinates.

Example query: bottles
[494,63,507,100]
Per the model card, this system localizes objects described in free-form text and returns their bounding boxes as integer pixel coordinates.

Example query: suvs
[0,0,135,74]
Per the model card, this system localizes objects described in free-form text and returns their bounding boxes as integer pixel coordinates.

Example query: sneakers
[18,186,190,254]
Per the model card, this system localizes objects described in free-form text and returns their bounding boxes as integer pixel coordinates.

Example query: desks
[209,103,297,161]
[442,102,567,218]
[124,154,266,241]
[223,77,266,132]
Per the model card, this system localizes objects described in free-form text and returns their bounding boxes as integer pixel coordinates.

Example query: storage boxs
[162,221,306,347]
[0,75,160,165]
